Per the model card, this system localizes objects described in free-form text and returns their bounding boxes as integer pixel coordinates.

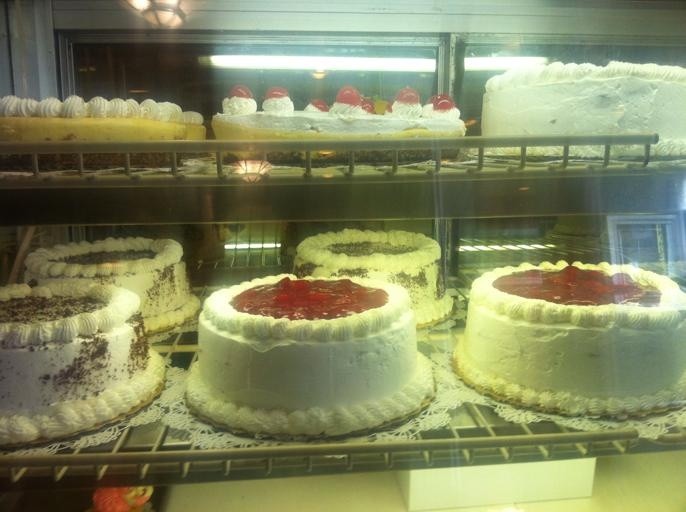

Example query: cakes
[452,259,684,421]
[23,237,201,333]
[213,85,467,160]
[1,279,166,446]
[292,227,454,326]
[468,60,684,158]
[1,94,206,140]
[184,273,433,436]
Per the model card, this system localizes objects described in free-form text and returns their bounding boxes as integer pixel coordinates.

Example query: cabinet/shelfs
[0,133,686,490]
